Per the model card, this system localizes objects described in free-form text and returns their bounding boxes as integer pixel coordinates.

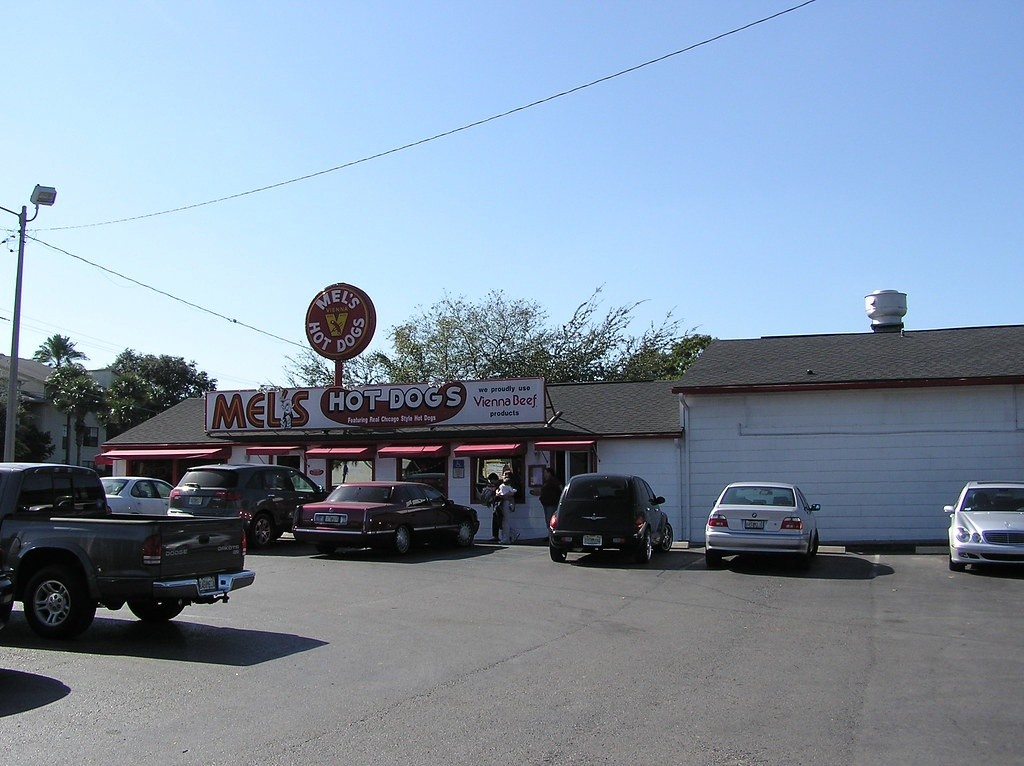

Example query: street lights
[3,184,57,464]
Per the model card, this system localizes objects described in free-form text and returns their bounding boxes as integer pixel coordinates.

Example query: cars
[548,473,674,566]
[97,477,175,520]
[290,481,480,558]
[943,482,1024,570]
[704,482,822,568]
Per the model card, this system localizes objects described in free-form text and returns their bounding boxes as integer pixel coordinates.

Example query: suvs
[164,463,333,551]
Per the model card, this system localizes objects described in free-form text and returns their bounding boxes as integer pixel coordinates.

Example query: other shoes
[498,539,510,545]
[512,533,520,544]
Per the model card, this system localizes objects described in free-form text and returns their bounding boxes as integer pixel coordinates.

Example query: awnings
[378,444,447,458]
[454,443,526,458]
[306,446,373,460]
[95,448,231,465]
[246,445,309,466]
[535,441,600,463]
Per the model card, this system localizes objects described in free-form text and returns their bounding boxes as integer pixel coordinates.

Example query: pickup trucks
[0,463,255,641]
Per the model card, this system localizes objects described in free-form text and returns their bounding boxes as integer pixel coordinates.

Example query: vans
[406,473,480,499]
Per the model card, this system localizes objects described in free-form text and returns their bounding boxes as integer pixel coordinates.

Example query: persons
[488,471,520,544]
[540,468,562,541]
